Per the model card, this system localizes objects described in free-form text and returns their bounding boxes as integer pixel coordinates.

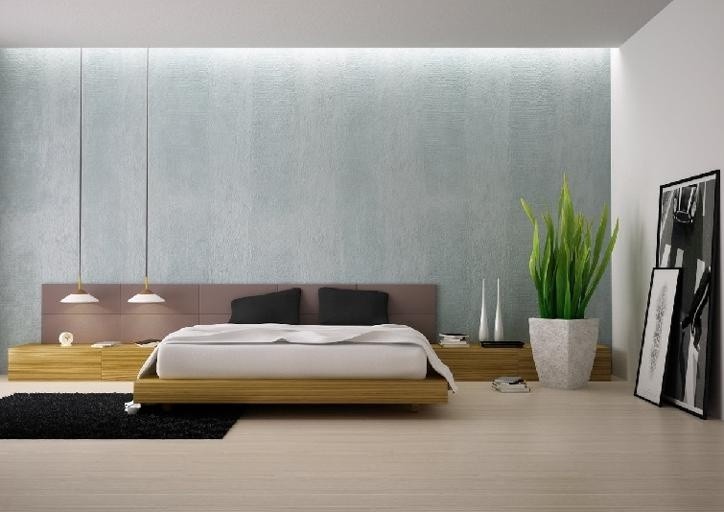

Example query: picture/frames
[651,169,720,418]
[632,268,683,406]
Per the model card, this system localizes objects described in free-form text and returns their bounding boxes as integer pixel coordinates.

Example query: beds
[132,319,451,416]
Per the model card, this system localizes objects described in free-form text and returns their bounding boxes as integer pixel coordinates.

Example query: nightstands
[440,340,612,380]
[7,342,156,382]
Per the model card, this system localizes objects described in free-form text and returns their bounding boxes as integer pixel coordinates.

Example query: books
[134,338,161,348]
[94,340,120,346]
[492,375,532,394]
[438,332,475,349]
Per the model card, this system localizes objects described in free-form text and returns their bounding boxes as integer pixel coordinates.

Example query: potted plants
[519,175,621,389]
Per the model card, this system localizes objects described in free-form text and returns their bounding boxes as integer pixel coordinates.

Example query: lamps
[57,48,102,303]
[126,47,168,305]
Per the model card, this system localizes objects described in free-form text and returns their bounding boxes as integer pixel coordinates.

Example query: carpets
[0,391,238,439]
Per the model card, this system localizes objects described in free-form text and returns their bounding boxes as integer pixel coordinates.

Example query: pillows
[228,287,301,325]
[318,287,394,325]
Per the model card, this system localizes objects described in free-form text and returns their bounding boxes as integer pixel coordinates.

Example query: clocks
[59,332,73,346]
